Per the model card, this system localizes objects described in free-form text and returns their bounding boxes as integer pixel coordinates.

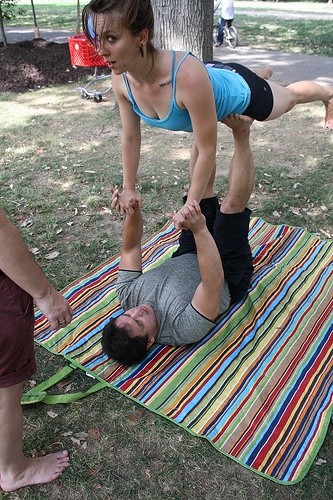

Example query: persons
[101,114,254,365]
[0,211,71,492]
[213,0,234,48]
[82,0,333,227]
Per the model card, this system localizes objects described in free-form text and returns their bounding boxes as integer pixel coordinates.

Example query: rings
[59,320,66,324]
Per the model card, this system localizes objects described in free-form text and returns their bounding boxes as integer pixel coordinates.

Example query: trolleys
[68,33,113,103]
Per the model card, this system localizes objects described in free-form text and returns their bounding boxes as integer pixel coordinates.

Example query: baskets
[68,33,109,67]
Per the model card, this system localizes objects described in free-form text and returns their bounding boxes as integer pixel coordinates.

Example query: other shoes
[215,42,222,47]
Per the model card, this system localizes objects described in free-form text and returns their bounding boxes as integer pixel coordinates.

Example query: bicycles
[211,10,238,49]
